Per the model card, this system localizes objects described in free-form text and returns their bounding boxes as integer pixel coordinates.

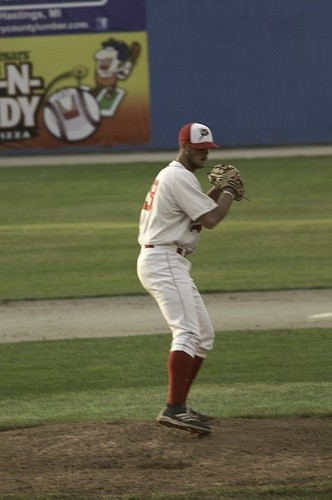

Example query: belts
[142,245,185,257]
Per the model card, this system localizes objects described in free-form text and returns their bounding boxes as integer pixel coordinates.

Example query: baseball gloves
[207,163,246,201]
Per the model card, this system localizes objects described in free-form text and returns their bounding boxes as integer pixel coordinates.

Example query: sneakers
[189,408,216,424]
[156,407,212,434]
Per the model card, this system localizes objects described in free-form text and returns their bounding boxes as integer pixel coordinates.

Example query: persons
[137,123,245,434]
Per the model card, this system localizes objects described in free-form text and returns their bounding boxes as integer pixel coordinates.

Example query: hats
[178,123,220,149]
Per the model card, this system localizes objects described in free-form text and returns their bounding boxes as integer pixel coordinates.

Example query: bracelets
[223,190,235,200]
